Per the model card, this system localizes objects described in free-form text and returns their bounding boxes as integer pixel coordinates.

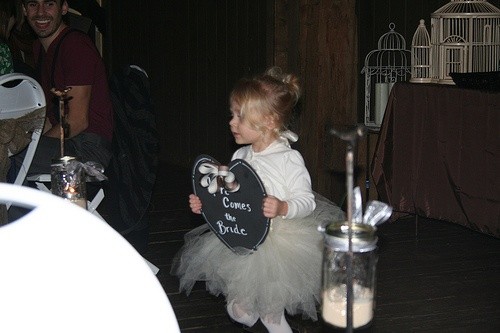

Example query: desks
[0,183,181,332]
[372,80,500,238]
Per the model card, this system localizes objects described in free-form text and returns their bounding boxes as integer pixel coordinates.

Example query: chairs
[0,72,46,211]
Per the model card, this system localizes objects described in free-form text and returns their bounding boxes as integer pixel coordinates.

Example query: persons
[189,67,316,333]
[0,0,115,223]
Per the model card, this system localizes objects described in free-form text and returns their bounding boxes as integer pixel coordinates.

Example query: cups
[317,219,378,330]
[50,161,88,210]
